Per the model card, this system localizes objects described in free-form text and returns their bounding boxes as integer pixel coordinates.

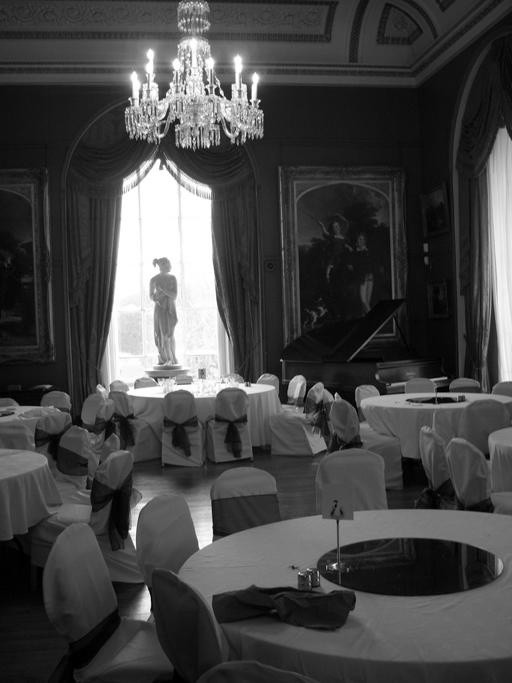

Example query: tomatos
[280,299,453,394]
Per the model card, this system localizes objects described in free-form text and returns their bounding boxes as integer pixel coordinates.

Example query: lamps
[146,568,239,682]
[256,372,280,395]
[30,450,145,584]
[405,377,437,393]
[161,390,206,467]
[80,393,113,450]
[134,376,159,389]
[269,381,328,457]
[51,426,90,498]
[174,375,192,385]
[210,466,281,541]
[136,495,199,596]
[225,374,243,382]
[40,391,72,409]
[110,380,128,393]
[354,384,380,422]
[206,387,255,464]
[0,397,19,406]
[316,448,388,515]
[42,522,179,682]
[413,426,457,510]
[449,378,481,393]
[282,374,307,413]
[329,398,404,491]
[31,411,72,461]
[108,390,162,462]
[447,438,494,512]
[197,661,321,683]
[492,381,512,397]
[96,384,108,399]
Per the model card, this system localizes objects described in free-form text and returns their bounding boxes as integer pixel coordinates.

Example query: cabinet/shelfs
[297,570,312,592]
[307,567,320,588]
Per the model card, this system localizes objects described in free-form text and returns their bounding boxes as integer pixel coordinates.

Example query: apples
[250,71,259,100]
[147,50,154,84]
[206,57,216,86]
[130,71,140,102]
[172,57,181,91]
[237,63,244,98]
[235,55,242,87]
[189,39,199,69]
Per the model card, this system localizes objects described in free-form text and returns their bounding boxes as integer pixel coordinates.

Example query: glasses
[124,0,265,154]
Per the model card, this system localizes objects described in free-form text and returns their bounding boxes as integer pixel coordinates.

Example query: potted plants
[278,164,412,350]
[1,168,56,365]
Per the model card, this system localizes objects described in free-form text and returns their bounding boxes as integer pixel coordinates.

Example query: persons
[148,256,179,365]
[348,232,379,317]
[301,211,357,286]
